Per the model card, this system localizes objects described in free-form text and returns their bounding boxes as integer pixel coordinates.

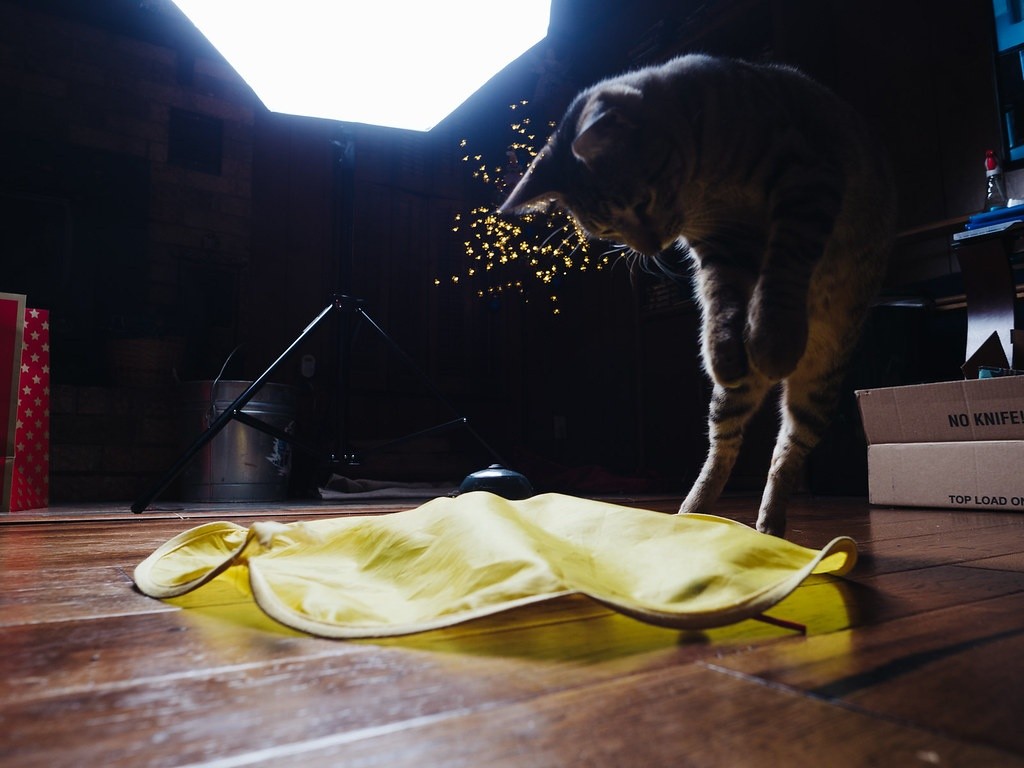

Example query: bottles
[984,150,1009,210]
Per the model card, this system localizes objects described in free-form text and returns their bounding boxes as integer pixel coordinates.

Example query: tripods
[130,136,510,516]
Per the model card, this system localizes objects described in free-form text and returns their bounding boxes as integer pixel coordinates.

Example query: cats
[485,45,905,540]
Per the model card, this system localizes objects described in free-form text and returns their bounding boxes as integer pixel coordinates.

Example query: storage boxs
[854,328,1024,512]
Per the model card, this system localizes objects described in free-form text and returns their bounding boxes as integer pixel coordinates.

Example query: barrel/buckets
[174,342,302,504]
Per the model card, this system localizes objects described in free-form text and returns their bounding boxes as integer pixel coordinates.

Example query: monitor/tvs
[986,0,1024,172]
